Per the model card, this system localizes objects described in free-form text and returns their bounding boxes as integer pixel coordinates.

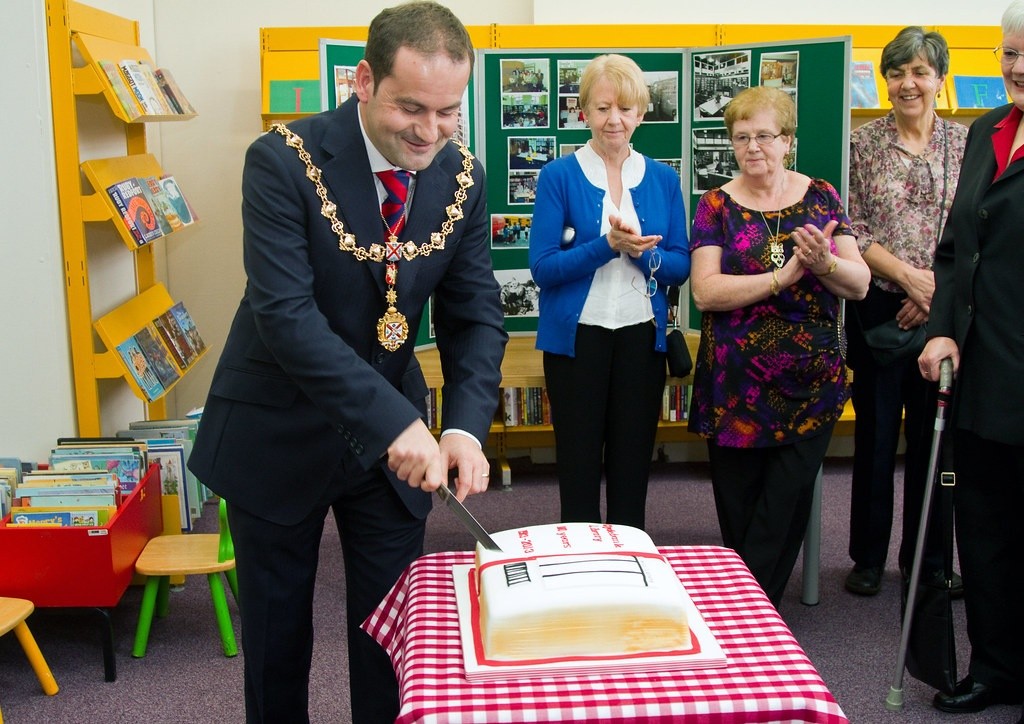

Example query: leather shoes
[934,673,1024,713]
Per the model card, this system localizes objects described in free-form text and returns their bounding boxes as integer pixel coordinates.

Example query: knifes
[437,484,501,551]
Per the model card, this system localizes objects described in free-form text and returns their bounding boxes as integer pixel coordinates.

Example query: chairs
[0,596,57,721]
[129,494,241,659]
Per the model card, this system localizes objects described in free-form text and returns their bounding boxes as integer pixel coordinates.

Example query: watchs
[818,256,837,277]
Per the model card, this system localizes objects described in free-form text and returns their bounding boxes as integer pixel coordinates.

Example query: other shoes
[845,562,885,593]
[950,573,964,598]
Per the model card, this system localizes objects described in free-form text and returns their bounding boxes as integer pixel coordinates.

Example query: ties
[375,171,410,269]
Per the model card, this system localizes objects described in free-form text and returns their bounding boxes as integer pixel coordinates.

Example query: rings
[924,371,930,374]
[629,243,635,251]
[804,249,812,256]
[482,474,491,477]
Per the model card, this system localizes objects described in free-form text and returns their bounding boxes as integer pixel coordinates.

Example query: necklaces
[742,172,785,268]
[268,119,474,353]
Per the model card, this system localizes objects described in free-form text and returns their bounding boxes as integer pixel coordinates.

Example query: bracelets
[770,267,783,295]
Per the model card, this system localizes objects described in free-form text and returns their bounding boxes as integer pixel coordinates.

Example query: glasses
[631,248,661,297]
[993,44,1024,64]
[731,131,785,145]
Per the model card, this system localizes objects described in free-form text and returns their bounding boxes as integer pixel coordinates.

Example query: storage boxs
[0,462,166,606]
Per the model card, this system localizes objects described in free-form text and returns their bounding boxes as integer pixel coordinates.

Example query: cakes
[468,522,701,669]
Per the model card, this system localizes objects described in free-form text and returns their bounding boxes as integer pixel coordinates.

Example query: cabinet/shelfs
[395,332,911,488]
[47,1,210,440]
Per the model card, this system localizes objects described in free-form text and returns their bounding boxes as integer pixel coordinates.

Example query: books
[106,172,196,245]
[99,56,186,123]
[850,61,880,108]
[953,75,1008,109]
[115,301,206,403]
[424,385,694,430]
[0,400,215,535]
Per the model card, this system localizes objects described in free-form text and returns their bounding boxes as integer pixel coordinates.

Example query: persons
[916,0,1024,713]
[841,23,971,600]
[528,52,693,534]
[690,85,871,611]
[563,67,578,84]
[713,157,724,174]
[566,105,583,124]
[499,277,533,317]
[181,1,510,724]
[502,64,553,245]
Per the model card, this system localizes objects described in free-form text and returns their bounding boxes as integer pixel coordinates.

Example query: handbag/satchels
[863,320,927,368]
[666,330,692,378]
[901,578,958,697]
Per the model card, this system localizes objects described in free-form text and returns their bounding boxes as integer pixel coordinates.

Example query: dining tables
[360,545,851,724]
